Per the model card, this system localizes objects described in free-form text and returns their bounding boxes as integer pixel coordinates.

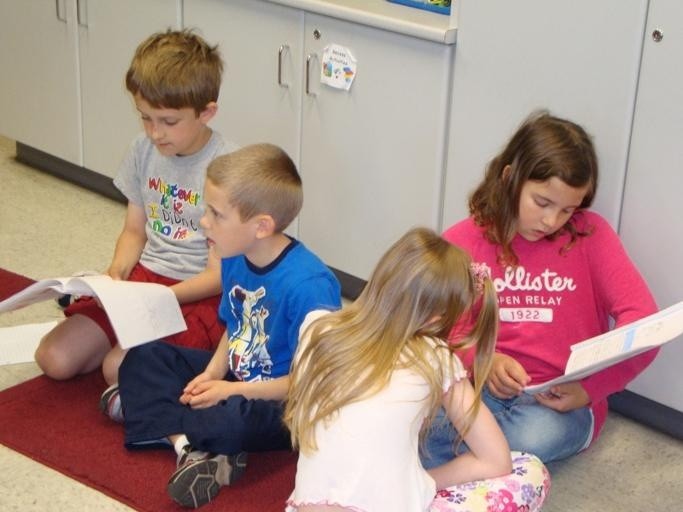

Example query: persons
[99,142,343,510]
[35,28,243,383]
[284,225,552,510]
[419,110,660,471]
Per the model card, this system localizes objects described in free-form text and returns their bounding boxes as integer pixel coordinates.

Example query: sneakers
[166,445,247,510]
[100,383,126,427]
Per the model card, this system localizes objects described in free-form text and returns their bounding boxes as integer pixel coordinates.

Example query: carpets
[0,270,301,511]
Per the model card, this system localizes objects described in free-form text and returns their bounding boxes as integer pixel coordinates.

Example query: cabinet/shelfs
[4,2,182,207]
[186,1,453,306]
[443,1,682,435]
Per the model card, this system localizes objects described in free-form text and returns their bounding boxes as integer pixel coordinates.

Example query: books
[0,274,188,349]
[523,301,683,395]
